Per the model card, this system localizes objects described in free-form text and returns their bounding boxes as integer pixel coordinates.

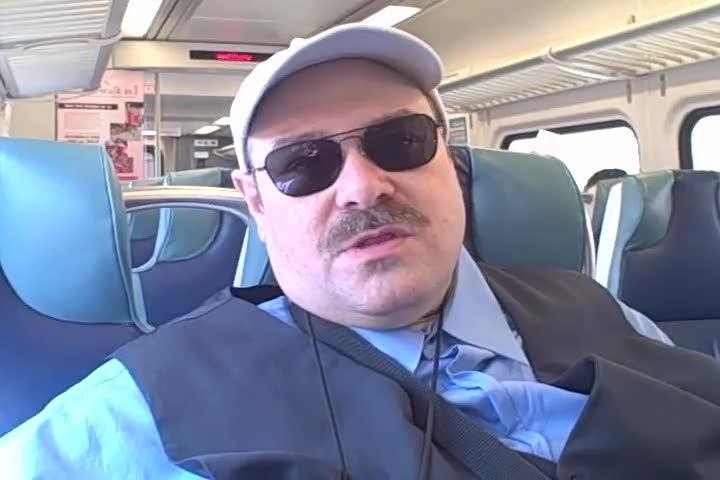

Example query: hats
[228,22,450,176]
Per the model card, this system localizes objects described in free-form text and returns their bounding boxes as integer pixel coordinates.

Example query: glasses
[248,113,445,197]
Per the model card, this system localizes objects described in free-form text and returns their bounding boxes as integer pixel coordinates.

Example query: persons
[0,18,719,480]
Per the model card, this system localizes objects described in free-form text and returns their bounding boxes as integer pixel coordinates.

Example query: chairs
[444,139,718,361]
[0,139,279,438]
